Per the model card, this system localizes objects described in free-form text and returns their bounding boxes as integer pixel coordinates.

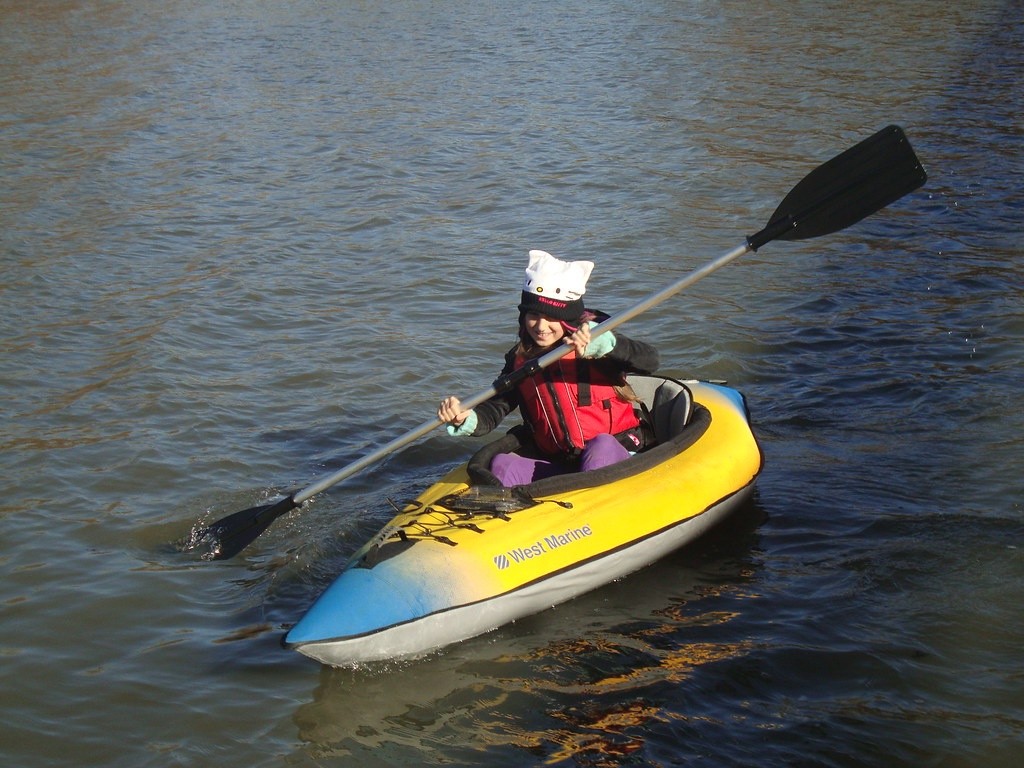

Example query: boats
[282,377,767,668]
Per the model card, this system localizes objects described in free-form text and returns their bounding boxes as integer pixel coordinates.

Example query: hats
[522,250,595,321]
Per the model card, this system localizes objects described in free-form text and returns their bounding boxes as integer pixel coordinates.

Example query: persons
[437,248,659,486]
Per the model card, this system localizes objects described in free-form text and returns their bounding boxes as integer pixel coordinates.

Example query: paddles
[171,122,931,562]
